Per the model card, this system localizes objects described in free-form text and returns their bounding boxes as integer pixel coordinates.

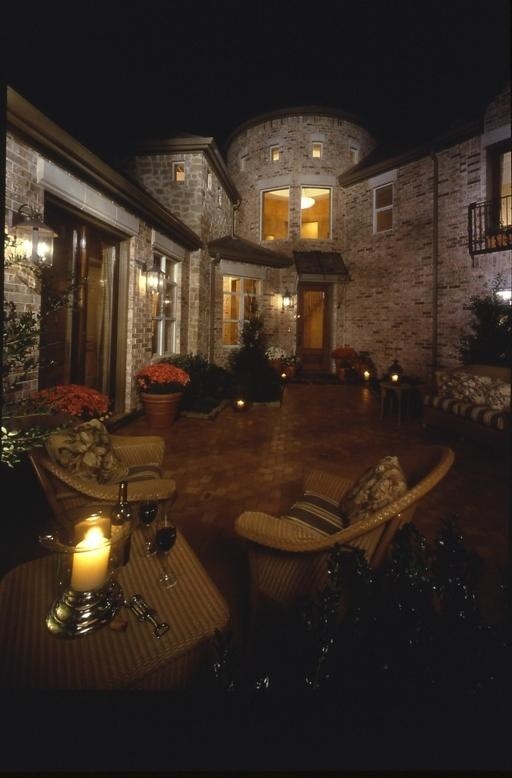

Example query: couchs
[28,418,175,542]
[415,366,512,448]
[233,447,455,602]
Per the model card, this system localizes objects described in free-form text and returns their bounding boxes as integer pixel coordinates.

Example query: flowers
[330,347,353,359]
[134,363,191,394]
[36,382,112,418]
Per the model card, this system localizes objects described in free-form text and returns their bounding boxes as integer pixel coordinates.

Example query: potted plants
[477,218,512,249]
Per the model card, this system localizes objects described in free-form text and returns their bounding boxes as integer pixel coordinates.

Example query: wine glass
[154,514,177,591]
[138,493,158,557]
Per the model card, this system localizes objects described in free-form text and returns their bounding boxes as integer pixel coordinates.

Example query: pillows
[435,368,512,412]
[44,418,129,486]
[338,456,408,528]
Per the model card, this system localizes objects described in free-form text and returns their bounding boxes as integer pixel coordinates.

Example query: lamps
[10,204,60,269]
[301,197,316,211]
[142,266,167,300]
[282,288,294,313]
[495,284,512,303]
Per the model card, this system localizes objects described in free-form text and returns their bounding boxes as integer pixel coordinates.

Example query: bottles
[109,480,134,567]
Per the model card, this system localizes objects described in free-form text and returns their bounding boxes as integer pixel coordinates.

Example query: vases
[139,393,183,426]
[335,359,347,372]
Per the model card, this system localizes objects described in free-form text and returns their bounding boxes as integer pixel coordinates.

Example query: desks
[378,381,411,426]
[0,522,232,693]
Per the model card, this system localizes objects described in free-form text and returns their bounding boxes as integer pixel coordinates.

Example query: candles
[70,528,111,593]
